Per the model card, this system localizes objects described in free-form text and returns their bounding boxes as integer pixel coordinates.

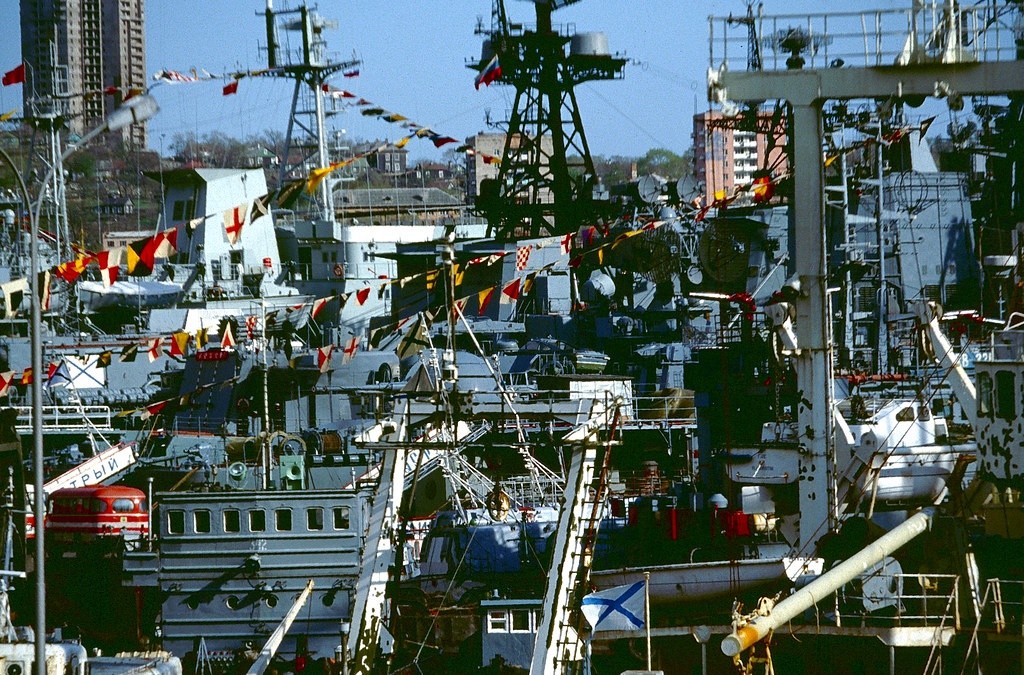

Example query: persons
[521,511,537,523]
[590,584,600,593]
[731,596,747,615]
[788,578,796,595]
[322,656,335,675]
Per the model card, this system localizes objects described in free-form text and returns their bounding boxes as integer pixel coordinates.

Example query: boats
[2,0,1024,674]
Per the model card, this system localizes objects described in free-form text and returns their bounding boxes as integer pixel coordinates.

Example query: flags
[2,62,26,86]
[0,66,938,445]
[475,53,502,90]
[580,578,645,633]
